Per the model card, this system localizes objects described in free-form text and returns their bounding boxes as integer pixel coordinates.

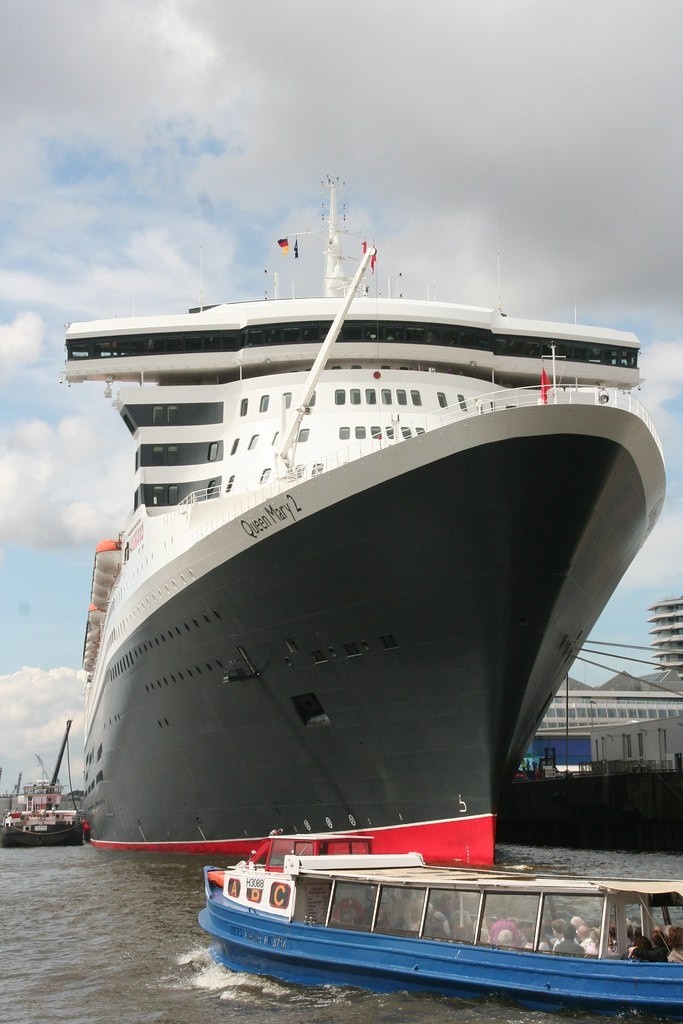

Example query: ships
[57,172,666,865]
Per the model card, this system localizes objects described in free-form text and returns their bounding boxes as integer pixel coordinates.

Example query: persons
[355,889,682,965]
[520,759,542,781]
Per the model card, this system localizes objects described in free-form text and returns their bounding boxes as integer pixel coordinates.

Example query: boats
[0,719,90,847]
[197,835,683,1019]
[82,539,122,672]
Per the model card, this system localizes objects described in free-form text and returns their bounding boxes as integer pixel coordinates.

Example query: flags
[540,367,552,405]
[293,239,298,258]
[277,238,288,256]
[371,246,376,274]
[362,241,367,253]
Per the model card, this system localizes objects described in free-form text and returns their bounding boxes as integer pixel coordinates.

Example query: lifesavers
[335,898,363,925]
